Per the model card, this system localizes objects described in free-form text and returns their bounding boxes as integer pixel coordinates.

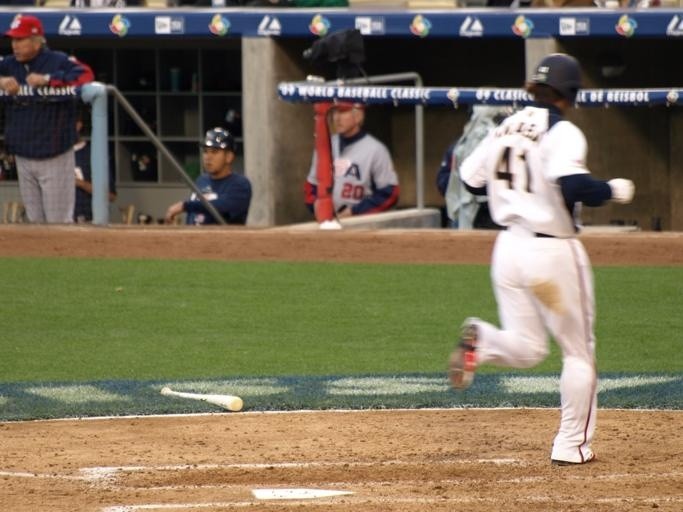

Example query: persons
[167,126,252,225]
[0,16,117,224]
[303,102,508,230]
[449,52,636,466]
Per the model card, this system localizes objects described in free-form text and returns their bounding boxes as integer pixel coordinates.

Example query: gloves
[606,177,637,207]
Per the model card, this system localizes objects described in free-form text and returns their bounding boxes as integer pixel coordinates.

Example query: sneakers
[551,451,596,466]
[446,320,481,392]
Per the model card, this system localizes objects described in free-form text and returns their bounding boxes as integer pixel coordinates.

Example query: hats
[197,127,237,152]
[330,100,366,111]
[0,15,43,39]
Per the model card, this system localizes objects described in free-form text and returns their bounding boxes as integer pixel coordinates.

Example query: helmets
[532,52,582,109]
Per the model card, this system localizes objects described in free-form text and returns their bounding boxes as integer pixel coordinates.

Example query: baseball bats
[159,386,243,412]
